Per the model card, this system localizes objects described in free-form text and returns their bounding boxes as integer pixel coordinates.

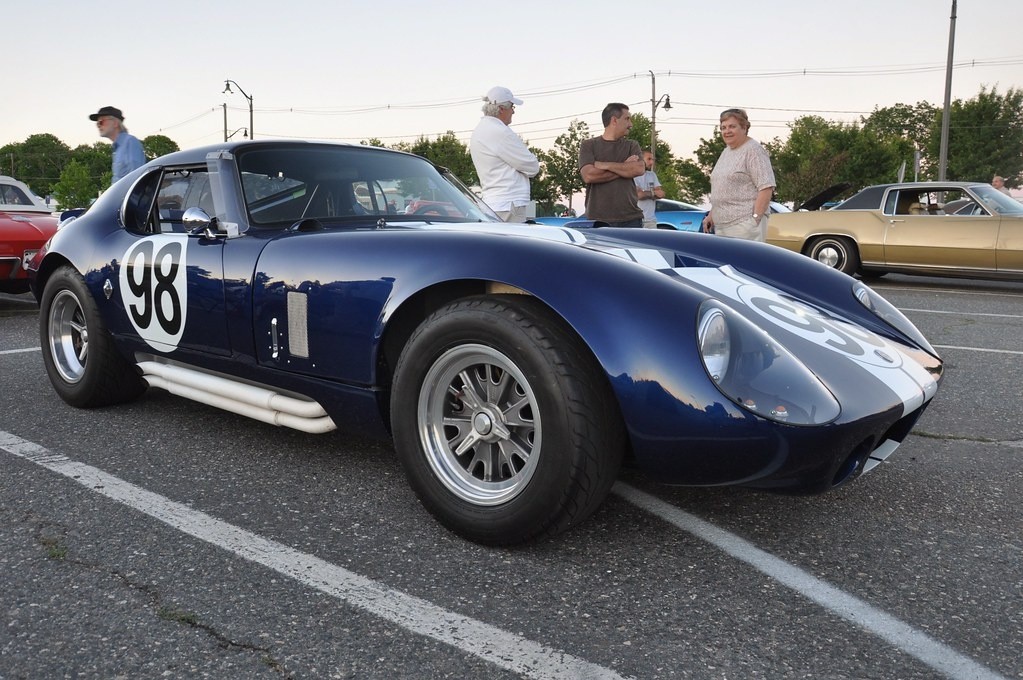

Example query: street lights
[221,79,254,143]
[222,104,248,144]
[649,94,672,175]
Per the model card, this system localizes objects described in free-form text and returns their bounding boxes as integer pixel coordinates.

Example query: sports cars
[26,140,944,548]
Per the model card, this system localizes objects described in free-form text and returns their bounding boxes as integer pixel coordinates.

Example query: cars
[0,176,74,294]
[523,197,718,235]
[770,180,1023,287]
[352,183,483,224]
[944,199,989,216]
[157,172,294,226]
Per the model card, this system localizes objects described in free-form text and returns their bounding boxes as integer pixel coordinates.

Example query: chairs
[928,203,946,215]
[908,202,930,215]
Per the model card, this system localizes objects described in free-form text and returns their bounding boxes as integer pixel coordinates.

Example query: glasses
[97,119,113,126]
[512,105,515,111]
[720,108,746,120]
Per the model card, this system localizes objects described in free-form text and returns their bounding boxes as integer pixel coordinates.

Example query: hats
[483,87,524,105]
[89,106,124,121]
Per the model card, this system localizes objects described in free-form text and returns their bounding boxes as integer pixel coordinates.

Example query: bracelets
[752,214,761,222]
[652,191,655,198]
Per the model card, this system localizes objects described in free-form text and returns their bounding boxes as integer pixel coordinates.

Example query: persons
[406,201,414,212]
[634,149,664,229]
[702,109,776,242]
[90,106,145,185]
[579,103,646,226]
[992,176,1011,196]
[384,200,396,211]
[470,86,539,223]
